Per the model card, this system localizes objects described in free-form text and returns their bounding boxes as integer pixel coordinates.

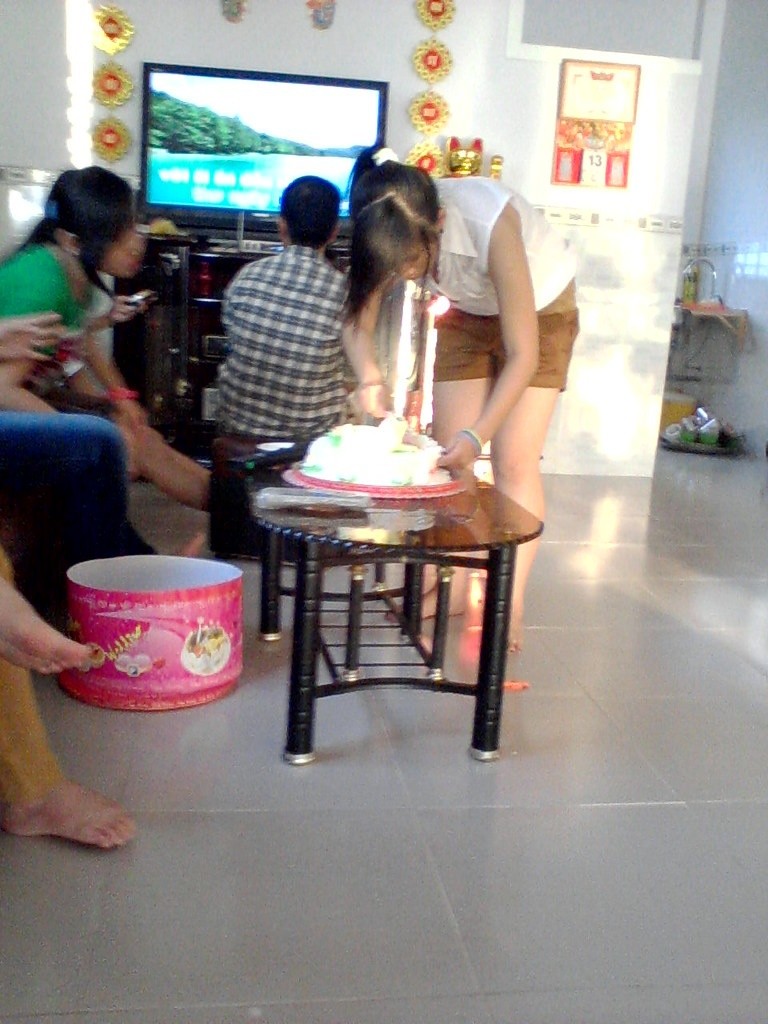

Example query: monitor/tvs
[134,62,389,231]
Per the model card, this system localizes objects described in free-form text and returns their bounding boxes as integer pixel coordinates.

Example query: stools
[209,436,343,568]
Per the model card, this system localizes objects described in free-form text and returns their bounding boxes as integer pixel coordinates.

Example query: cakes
[299,414,453,488]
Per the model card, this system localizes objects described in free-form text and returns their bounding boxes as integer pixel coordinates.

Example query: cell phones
[123,287,157,305]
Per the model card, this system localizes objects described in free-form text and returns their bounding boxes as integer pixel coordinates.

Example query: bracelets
[460,429,484,455]
[109,388,138,399]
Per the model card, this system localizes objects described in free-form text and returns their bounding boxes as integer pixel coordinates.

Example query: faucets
[682,257,716,299]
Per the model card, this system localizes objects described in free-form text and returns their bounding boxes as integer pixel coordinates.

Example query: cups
[666,423,681,443]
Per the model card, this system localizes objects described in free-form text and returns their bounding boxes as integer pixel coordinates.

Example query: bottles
[683,260,699,308]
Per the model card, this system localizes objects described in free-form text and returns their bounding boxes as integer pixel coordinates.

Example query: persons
[0,166,211,847]
[341,144,581,651]
[220,176,351,439]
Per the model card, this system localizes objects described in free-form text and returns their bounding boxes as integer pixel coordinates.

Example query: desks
[242,466,544,766]
